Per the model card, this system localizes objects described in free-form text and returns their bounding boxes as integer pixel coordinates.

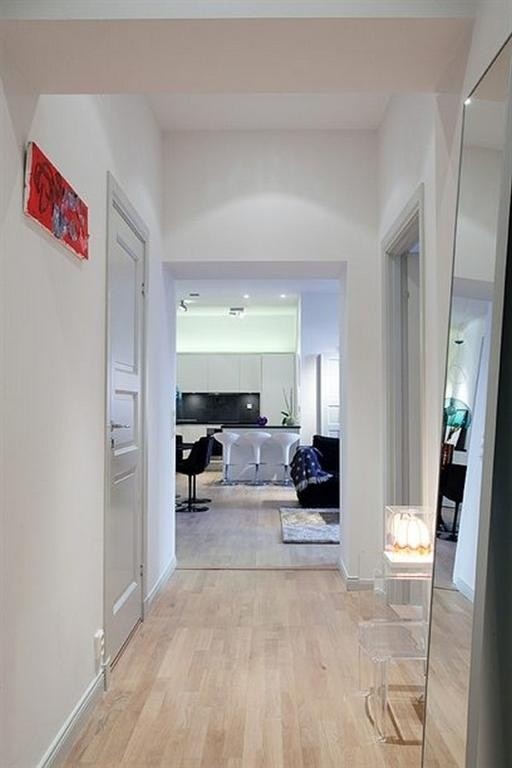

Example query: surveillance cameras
[455,338,464,344]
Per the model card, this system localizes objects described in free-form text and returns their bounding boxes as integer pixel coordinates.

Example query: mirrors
[422,33,512,768]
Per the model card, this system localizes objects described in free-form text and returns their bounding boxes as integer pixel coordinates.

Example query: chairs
[296,434,339,507]
[436,463,467,542]
[175,435,214,512]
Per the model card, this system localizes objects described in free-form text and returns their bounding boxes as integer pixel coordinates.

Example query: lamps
[228,307,245,316]
[178,300,187,312]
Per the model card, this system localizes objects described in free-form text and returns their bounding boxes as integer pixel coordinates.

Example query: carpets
[278,506,341,545]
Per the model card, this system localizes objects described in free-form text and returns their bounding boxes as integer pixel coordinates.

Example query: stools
[244,432,271,486]
[213,432,240,486]
[272,433,300,487]
